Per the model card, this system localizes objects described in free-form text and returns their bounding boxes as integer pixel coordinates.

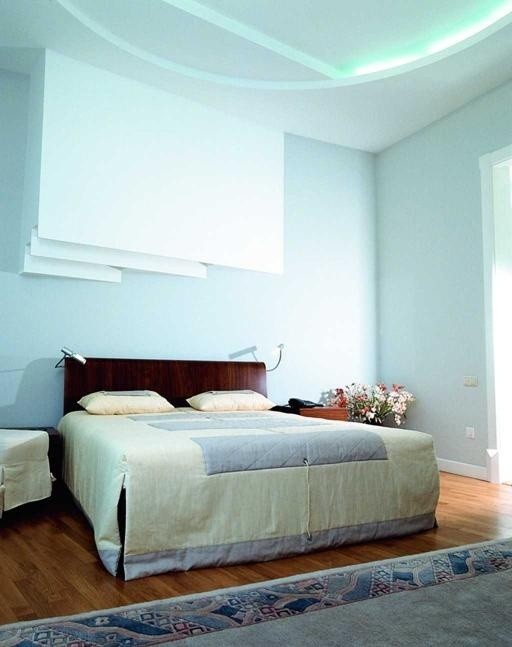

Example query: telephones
[288,398,315,408]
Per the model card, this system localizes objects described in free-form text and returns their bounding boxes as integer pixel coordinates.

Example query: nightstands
[1,425,64,503]
[289,404,348,422]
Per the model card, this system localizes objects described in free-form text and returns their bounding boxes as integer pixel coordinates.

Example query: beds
[56,356,439,581]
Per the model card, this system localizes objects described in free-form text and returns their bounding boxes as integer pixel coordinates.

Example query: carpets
[1,534,510,646]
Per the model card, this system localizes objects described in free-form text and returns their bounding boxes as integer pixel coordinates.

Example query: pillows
[76,389,176,415]
[186,389,276,412]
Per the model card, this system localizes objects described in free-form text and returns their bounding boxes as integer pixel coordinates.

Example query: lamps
[265,343,286,370]
[54,345,87,368]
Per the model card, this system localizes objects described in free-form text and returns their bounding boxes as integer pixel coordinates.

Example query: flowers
[326,379,416,426]
